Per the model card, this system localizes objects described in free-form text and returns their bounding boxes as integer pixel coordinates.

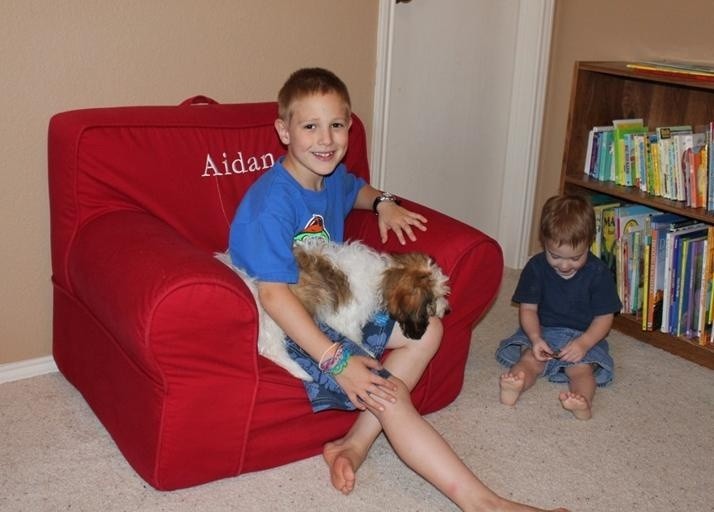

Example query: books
[584,118,714,346]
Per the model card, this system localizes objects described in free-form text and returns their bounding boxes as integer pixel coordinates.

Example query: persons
[500,194,622,419]
[229,68,567,512]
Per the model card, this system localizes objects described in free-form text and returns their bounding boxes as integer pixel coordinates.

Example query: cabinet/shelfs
[558,59,714,369]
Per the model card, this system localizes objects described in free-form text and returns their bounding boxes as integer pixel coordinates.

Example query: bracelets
[319,341,352,376]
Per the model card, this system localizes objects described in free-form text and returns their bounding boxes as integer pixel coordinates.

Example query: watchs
[373,192,399,214]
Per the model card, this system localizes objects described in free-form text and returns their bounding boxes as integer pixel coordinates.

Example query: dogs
[210,235,451,382]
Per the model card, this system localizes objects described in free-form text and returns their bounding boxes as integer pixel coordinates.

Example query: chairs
[42,94,505,491]
